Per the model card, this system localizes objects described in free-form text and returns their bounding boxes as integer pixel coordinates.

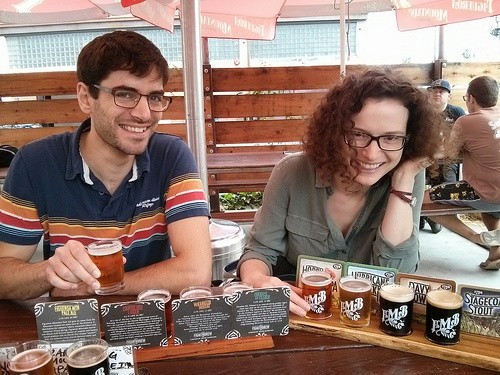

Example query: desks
[0,295,500,375]
[434,152,463,180]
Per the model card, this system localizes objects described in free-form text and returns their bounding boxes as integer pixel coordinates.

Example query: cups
[136,287,173,341]
[379,281,415,336]
[222,281,254,296]
[179,284,213,300]
[338,275,372,327]
[64,338,110,375]
[424,289,464,346]
[6,339,55,375]
[300,270,334,320]
[87,238,126,295]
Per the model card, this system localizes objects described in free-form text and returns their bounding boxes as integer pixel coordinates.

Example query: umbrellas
[0,0,500,218]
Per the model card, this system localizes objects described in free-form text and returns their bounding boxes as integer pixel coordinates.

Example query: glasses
[341,129,410,151]
[463,96,469,101]
[91,83,172,112]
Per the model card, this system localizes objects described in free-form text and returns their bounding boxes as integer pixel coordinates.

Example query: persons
[418,79,467,233]
[1,144,19,186]
[1,31,212,301]
[423,76,500,271]
[238,68,436,317]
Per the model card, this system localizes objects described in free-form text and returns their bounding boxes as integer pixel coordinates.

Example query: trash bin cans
[170,218,248,287]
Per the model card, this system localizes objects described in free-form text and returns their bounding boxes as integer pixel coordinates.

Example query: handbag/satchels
[0,145,19,167]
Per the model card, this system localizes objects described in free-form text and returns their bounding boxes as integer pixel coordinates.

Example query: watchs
[390,187,417,208]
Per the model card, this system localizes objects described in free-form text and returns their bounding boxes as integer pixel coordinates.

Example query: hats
[427,80,450,93]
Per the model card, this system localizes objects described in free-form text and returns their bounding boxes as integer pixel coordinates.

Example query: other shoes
[480,229,500,247]
[419,216,442,233]
[480,258,500,269]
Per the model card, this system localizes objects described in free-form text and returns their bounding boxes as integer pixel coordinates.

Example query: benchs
[420,201,477,218]
[208,150,286,226]
